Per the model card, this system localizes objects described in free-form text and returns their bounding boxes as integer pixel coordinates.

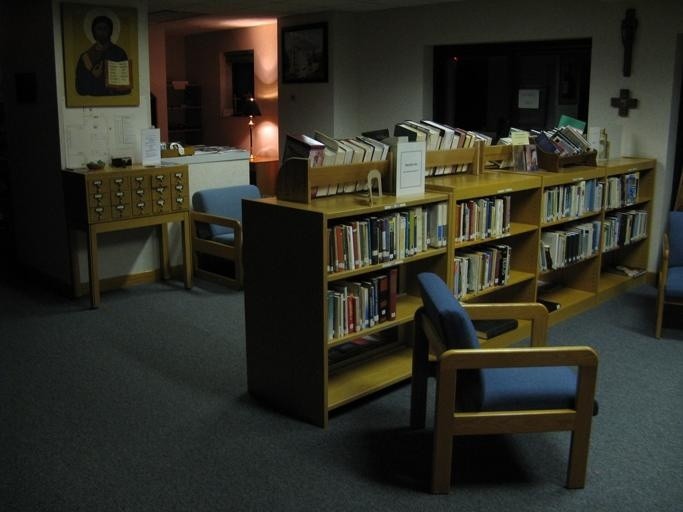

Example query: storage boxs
[165,74,204,148]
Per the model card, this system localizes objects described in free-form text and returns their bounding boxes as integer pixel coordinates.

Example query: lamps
[237,94,262,155]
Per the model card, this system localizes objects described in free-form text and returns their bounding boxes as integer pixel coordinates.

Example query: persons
[76,16,131,96]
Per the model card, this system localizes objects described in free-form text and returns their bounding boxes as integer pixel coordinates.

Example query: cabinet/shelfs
[58,155,197,310]
[248,155,282,199]
[239,134,543,429]
[500,136,657,333]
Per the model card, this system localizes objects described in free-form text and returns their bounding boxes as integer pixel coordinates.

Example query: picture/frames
[277,18,331,87]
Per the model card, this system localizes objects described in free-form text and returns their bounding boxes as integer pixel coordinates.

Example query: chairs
[178,180,266,291]
[402,263,610,500]
[651,208,680,339]
